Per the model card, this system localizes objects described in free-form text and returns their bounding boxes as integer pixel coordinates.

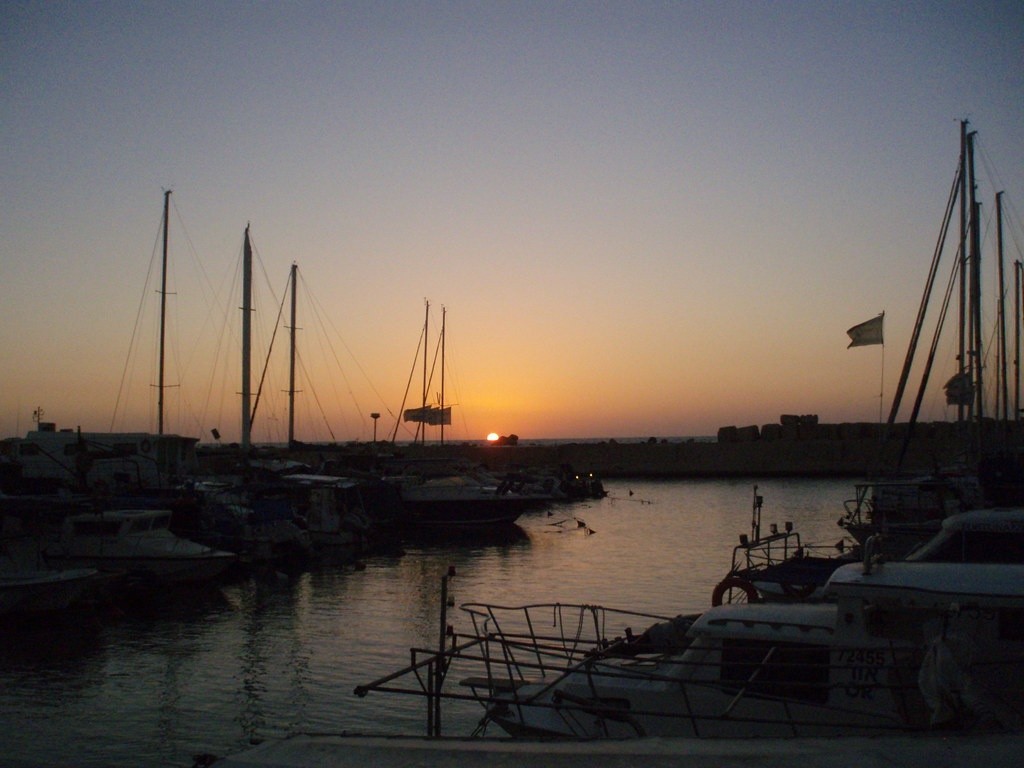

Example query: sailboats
[838,117,1023,562]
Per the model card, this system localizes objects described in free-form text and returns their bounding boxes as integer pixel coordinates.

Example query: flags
[847,313,885,349]
[404,407,452,425]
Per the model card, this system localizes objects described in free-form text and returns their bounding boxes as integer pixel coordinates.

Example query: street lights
[371,413,380,442]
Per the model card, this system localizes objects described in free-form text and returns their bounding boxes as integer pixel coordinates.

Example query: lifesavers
[711,578,759,607]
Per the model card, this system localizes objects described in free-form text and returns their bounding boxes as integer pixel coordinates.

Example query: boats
[713,485,1024,607]
[336,305,607,508]
[0,429,317,565]
[179,436,368,548]
[351,535,1024,737]
[0,535,99,617]
[369,475,535,526]
[0,510,239,586]
